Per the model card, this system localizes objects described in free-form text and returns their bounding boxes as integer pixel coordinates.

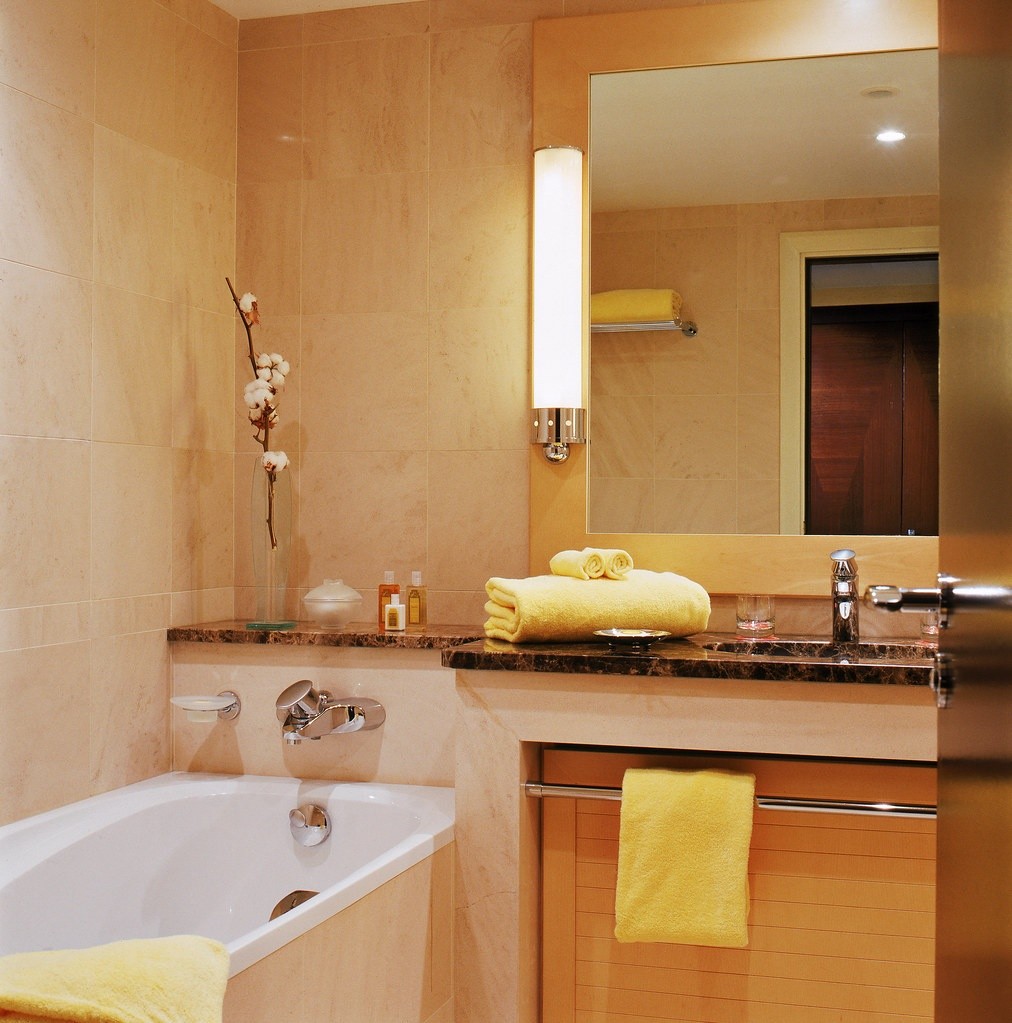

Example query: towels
[612,766,757,951]
[591,288,683,321]
[483,547,710,643]
[1,934,231,1023]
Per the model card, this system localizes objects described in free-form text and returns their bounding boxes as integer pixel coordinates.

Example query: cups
[735,594,775,639]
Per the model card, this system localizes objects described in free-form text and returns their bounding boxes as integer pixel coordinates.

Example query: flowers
[225,276,291,549]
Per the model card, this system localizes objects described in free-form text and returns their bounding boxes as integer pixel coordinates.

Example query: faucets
[276,678,387,748]
[830,549,860,639]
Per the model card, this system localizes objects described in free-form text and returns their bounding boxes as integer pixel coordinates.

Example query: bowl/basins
[305,577,363,632]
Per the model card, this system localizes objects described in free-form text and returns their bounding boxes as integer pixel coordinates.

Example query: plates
[593,628,671,651]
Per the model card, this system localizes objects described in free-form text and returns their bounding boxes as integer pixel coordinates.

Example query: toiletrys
[376,568,429,632]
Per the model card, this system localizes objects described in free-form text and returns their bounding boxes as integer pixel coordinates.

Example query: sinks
[701,639,938,662]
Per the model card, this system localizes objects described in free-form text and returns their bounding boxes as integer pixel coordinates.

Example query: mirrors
[521,0,940,595]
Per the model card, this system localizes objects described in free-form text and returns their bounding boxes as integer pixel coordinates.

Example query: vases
[247,456,293,619]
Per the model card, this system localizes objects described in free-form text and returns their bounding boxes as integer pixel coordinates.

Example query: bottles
[378,569,400,629]
[384,594,406,631]
[406,571,428,627]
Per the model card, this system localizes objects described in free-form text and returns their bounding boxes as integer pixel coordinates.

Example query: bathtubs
[0,771,458,1023]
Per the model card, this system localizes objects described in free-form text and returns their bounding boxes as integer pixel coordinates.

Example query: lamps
[531,142,586,464]
